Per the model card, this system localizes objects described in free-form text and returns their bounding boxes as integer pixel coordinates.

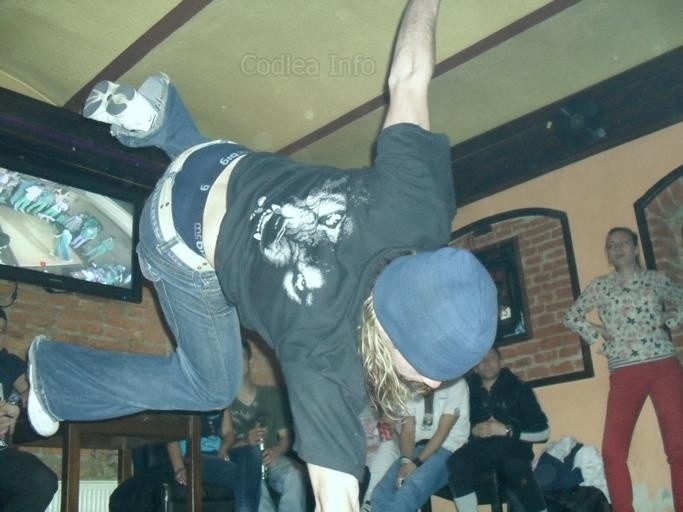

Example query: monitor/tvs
[0,153,143,304]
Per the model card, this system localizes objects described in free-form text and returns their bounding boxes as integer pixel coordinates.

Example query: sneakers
[27,335,60,437]
[83,80,155,132]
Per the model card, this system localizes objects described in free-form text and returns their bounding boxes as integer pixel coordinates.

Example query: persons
[110,339,550,512]
[0,168,127,287]
[0,309,58,512]
[27,0,498,512]
[561,229,683,512]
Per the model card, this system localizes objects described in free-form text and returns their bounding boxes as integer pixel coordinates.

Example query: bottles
[256,437,269,484]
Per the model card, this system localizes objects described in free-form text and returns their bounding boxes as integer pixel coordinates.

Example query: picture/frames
[469,235,535,348]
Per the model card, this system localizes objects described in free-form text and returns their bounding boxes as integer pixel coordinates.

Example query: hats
[373,247,499,382]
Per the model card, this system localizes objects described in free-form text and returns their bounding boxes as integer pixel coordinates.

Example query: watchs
[6,392,22,408]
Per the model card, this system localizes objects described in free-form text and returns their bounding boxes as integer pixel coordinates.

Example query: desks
[6,406,202,510]
